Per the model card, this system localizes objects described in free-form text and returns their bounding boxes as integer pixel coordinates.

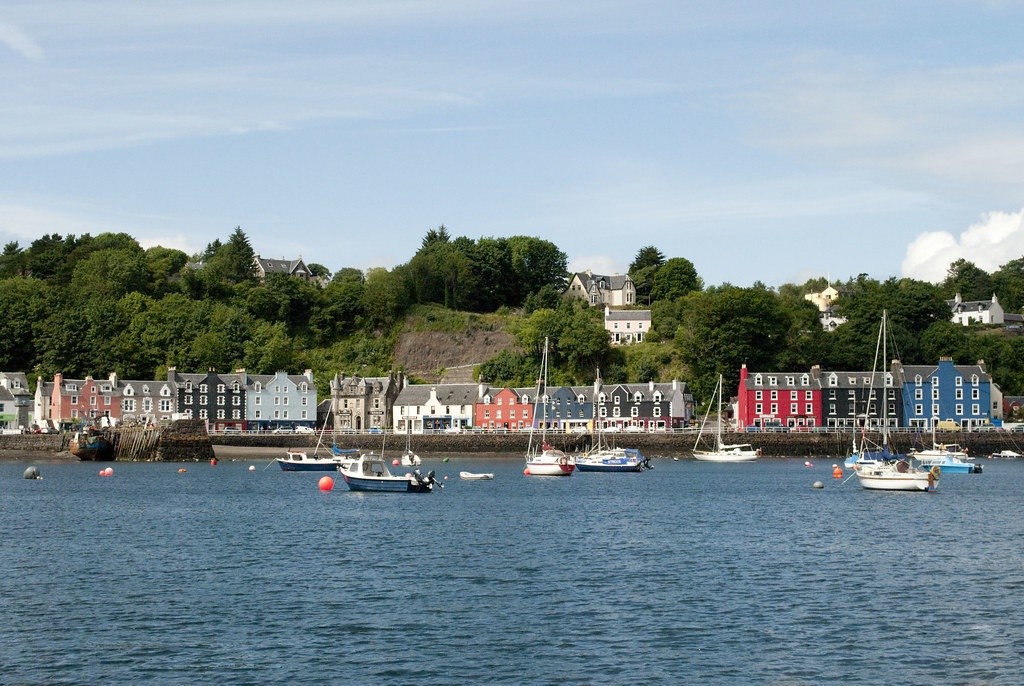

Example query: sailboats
[690,372,759,462]
[573,364,647,473]
[522,335,577,477]
[844,308,984,491]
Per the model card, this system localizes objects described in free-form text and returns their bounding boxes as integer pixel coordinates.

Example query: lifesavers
[932,465,941,476]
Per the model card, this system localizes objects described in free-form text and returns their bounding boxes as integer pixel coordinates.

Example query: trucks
[937,420,962,433]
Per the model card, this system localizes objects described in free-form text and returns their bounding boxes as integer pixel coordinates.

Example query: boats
[274,397,358,471]
[69,429,115,461]
[460,471,494,481]
[987,450,1024,459]
[401,400,422,467]
[332,441,360,456]
[337,397,434,493]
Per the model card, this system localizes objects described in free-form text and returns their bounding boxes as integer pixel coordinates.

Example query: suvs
[272,426,293,435]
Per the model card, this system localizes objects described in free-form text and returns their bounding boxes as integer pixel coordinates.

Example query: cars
[367,427,384,434]
[33,428,41,434]
[41,427,59,435]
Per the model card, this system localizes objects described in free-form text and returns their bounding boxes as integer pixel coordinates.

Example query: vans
[224,427,241,435]
[295,425,314,434]
